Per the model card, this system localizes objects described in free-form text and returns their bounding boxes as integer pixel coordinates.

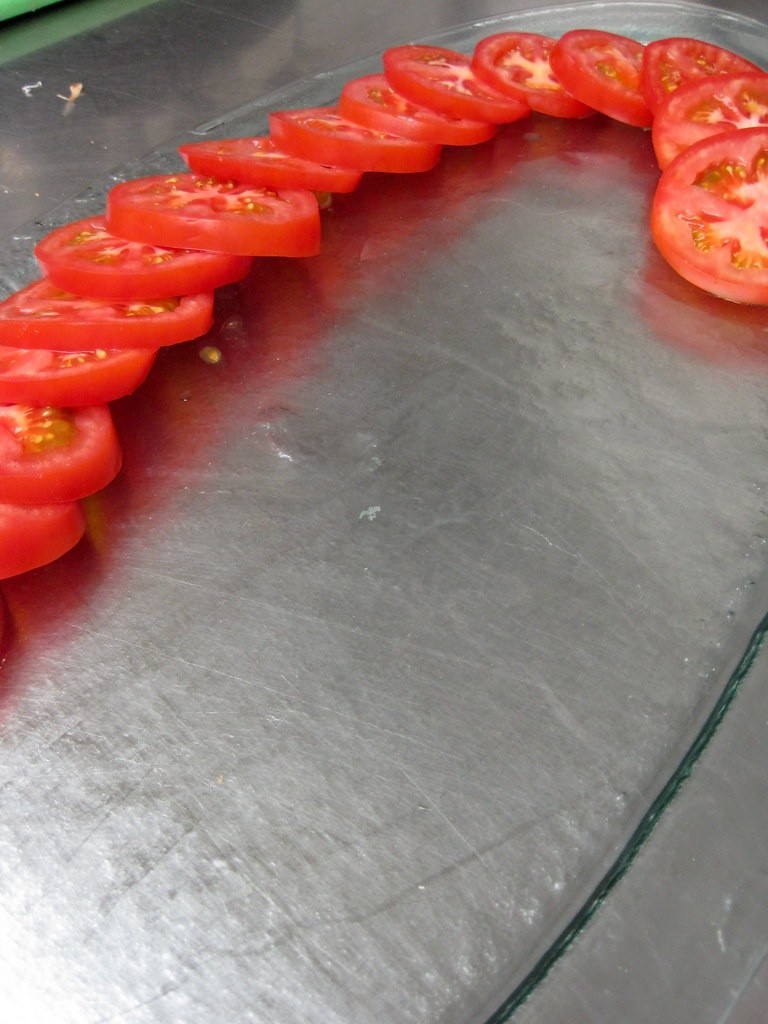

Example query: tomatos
[0,29,768,583]
